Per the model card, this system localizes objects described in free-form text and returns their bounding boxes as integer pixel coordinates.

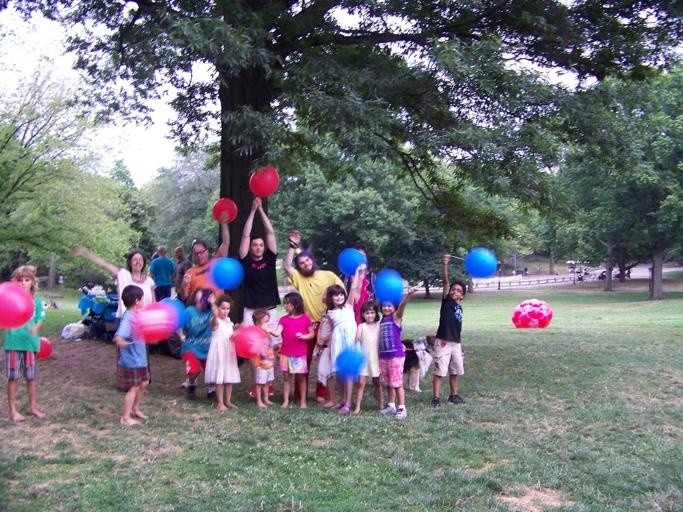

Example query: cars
[598,271,606,280]
[611,267,620,279]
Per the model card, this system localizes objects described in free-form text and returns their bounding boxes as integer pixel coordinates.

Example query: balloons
[247,164,280,198]
[0,281,34,327]
[159,297,186,331]
[36,337,50,359]
[130,302,175,343]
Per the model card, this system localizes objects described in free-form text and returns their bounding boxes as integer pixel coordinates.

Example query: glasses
[191,249,207,258]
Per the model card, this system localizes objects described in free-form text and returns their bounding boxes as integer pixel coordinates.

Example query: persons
[148,246,176,302]
[173,220,230,397]
[110,284,149,425]
[265,246,423,420]
[431,252,466,405]
[242,309,276,408]
[284,228,348,404]
[463,246,500,277]
[204,293,241,410]
[3,265,44,422]
[238,196,282,398]
[70,243,157,390]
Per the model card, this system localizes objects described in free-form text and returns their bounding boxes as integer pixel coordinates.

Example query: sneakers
[268,384,274,397]
[249,386,264,398]
[394,406,408,421]
[447,393,465,403]
[206,390,217,400]
[182,377,197,387]
[379,403,397,416]
[186,386,197,397]
[431,397,441,407]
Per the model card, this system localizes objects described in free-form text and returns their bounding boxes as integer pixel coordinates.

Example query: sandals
[339,403,353,415]
[331,399,345,410]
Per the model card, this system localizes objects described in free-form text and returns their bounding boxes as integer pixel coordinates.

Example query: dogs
[400,335,437,393]
